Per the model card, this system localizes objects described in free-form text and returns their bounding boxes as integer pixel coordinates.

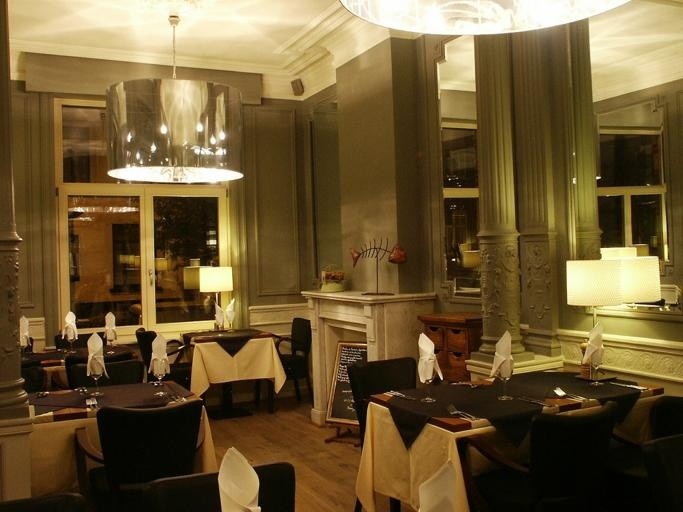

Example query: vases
[320,285,346,293]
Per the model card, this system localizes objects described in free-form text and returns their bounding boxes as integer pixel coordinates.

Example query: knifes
[609,382,649,390]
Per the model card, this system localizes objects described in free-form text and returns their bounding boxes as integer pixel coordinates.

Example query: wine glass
[214,315,225,334]
[227,313,237,333]
[90,364,105,398]
[107,329,115,354]
[589,349,605,386]
[154,362,166,395]
[67,329,78,354]
[20,336,29,361]
[419,357,437,403]
[497,360,514,401]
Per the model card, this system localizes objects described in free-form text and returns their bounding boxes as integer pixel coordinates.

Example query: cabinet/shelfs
[418,310,482,378]
[300,287,438,430]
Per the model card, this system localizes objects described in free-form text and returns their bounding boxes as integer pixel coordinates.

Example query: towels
[215,303,224,326]
[20,315,30,347]
[62,311,78,341]
[489,329,515,379]
[582,321,605,367]
[103,312,118,344]
[148,334,170,375]
[226,298,235,322]
[418,333,444,384]
[219,446,262,512]
[86,331,110,380]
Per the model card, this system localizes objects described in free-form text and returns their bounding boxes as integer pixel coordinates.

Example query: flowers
[320,265,346,282]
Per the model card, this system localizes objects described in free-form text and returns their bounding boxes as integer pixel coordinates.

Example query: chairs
[609,395,682,511]
[21,335,34,357]
[142,461,297,512]
[0,494,91,512]
[255,317,315,412]
[66,360,145,384]
[469,400,621,512]
[349,358,419,512]
[75,399,205,511]
[135,325,192,390]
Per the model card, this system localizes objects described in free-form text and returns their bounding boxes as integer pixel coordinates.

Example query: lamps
[105,21,245,185]
[134,256,141,269]
[183,266,201,303]
[462,251,480,271]
[154,258,167,281]
[190,257,201,265]
[565,256,662,380]
[119,255,127,265]
[338,0,637,34]
[199,267,234,333]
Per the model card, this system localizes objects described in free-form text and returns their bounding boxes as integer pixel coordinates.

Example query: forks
[553,387,586,402]
[85,399,98,410]
[451,382,483,388]
[446,405,475,423]
